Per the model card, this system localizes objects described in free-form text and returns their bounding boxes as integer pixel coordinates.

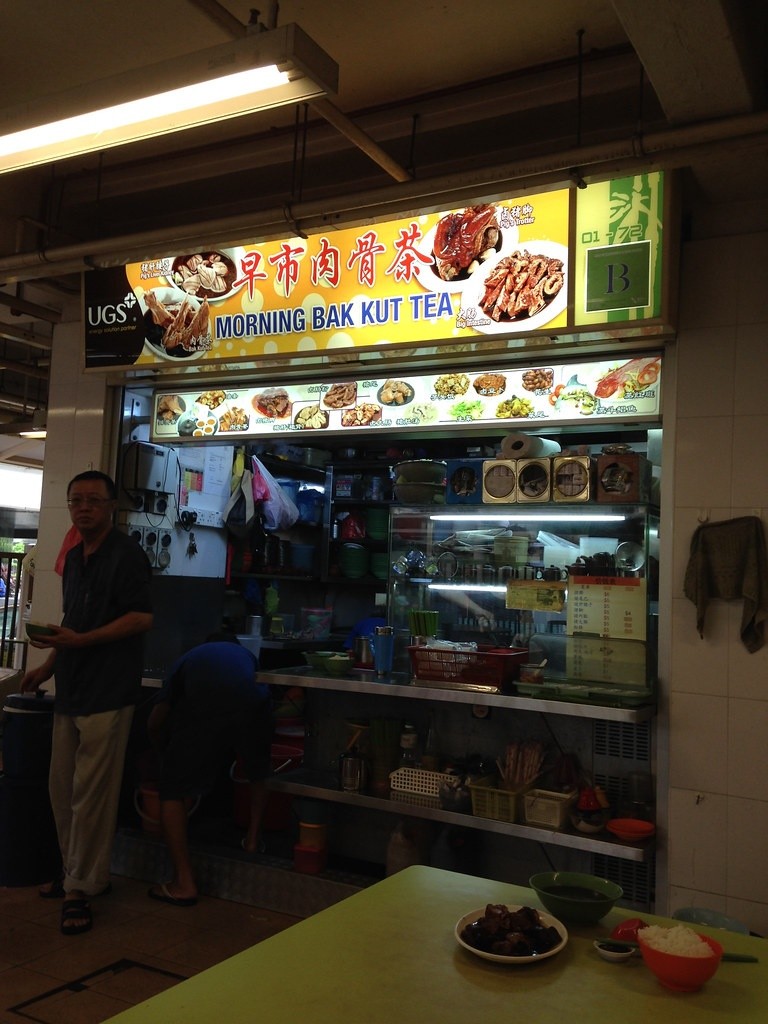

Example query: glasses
[64,495,115,509]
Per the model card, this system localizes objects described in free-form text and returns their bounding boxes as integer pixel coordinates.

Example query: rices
[637,923,715,957]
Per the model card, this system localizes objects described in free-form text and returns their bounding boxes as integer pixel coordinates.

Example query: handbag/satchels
[222,450,300,542]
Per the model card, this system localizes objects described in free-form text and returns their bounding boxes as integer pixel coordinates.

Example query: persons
[146,641,276,906]
[20,472,155,934]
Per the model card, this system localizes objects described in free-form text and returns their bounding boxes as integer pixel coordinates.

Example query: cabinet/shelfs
[231,450,399,583]
[257,667,654,861]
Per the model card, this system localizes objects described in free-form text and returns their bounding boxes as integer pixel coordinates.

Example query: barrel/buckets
[230,743,304,828]
[135,782,202,837]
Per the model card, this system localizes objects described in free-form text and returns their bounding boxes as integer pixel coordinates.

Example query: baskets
[388,767,460,798]
[391,790,445,811]
[466,764,557,822]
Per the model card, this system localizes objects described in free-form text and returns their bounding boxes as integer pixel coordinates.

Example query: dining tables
[100,864,768,1024]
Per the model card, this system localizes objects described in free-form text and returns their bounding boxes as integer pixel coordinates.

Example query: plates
[606,818,655,841]
[593,939,637,962]
[455,905,568,964]
[153,359,666,437]
[410,209,517,293]
[459,241,580,334]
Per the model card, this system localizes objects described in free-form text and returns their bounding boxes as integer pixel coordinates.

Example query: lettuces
[450,400,485,419]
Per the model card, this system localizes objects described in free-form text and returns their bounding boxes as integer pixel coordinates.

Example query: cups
[338,747,374,790]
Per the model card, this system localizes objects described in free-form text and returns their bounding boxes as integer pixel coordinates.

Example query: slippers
[37,879,66,900]
[146,883,200,907]
[59,897,93,935]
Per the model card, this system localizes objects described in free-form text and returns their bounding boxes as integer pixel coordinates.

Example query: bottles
[398,723,417,767]
[375,626,395,676]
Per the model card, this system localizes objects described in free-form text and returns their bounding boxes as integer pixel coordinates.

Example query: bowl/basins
[135,287,211,361]
[300,650,352,676]
[637,926,722,993]
[529,870,623,925]
[392,460,446,482]
[236,504,397,581]
[564,808,612,834]
[163,246,244,302]
[25,622,57,643]
[392,484,446,504]
